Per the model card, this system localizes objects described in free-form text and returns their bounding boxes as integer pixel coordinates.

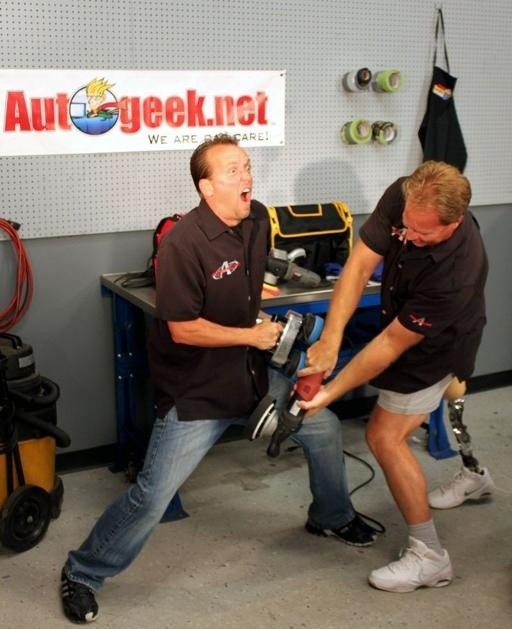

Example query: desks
[97,255,457,526]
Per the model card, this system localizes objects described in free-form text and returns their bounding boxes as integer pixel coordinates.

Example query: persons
[292,161,498,597]
[58,136,379,626]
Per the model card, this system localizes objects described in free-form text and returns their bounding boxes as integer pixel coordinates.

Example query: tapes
[341,118,396,145]
[345,68,404,94]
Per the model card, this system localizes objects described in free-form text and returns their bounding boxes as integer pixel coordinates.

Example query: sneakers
[368,533,453,594]
[428,465,496,510]
[304,514,379,547]
[60,566,100,625]
